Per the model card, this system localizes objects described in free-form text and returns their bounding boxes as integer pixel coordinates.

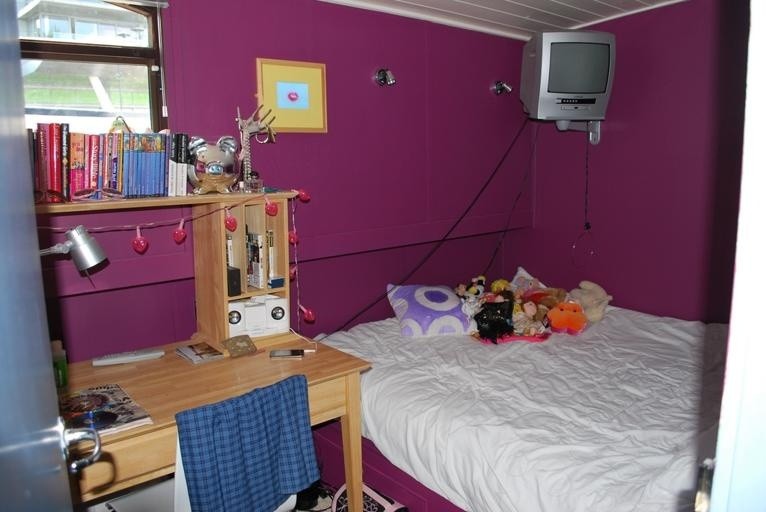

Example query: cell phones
[270,349,304,357]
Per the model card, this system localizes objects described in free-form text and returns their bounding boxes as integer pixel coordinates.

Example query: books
[266,230,274,280]
[58,382,154,445]
[247,234,264,290]
[226,235,234,267]
[27,121,187,203]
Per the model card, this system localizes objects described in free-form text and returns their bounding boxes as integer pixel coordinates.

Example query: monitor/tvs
[519,30,617,120]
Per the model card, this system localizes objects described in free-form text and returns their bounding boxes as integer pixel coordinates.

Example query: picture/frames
[254,57,330,135]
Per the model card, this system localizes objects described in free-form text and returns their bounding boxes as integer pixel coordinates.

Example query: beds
[310,303,731,511]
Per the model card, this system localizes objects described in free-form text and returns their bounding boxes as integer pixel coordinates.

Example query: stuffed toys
[455,274,616,347]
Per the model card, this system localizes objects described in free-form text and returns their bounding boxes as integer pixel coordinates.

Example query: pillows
[383,280,476,339]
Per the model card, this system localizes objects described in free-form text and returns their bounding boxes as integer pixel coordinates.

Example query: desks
[57,337,371,512]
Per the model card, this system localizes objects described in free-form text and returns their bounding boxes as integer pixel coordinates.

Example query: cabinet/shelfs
[191,198,290,358]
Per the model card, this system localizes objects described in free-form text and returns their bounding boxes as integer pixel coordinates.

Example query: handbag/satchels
[332,480,408,512]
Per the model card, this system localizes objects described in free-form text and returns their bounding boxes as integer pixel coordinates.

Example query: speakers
[228,302,245,338]
[265,299,289,336]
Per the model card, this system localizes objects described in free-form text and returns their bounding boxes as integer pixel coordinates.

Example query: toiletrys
[242,171,263,192]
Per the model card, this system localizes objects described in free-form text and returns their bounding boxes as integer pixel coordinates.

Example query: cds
[65,411,118,433]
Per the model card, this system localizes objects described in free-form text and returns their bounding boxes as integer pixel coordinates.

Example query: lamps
[377,70,395,87]
[37,224,110,278]
[495,80,513,95]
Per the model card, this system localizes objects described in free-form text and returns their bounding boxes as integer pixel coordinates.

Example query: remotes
[91,349,165,367]
